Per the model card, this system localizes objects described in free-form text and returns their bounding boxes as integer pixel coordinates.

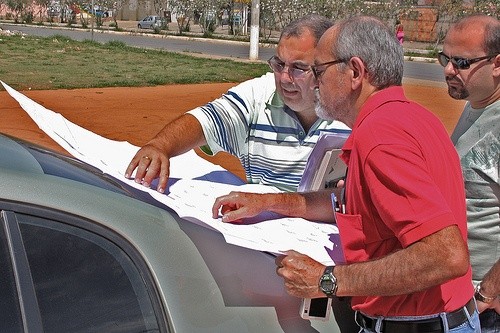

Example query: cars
[137,15,168,30]
[230,16,241,24]
[46,2,109,20]
[0,132,359,333]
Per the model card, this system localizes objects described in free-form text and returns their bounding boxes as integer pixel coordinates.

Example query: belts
[353,297,475,333]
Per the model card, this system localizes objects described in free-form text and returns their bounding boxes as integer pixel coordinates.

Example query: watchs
[318,266,338,298]
[475,282,497,304]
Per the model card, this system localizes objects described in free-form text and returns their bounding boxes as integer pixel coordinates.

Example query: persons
[125,16,353,194]
[211,16,481,333]
[437,15,500,333]
[394,20,404,44]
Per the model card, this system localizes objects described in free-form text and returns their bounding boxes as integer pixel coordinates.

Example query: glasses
[438,50,500,69]
[308,59,351,80]
[266,55,311,78]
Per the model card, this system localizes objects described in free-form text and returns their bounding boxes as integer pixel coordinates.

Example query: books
[310,148,348,191]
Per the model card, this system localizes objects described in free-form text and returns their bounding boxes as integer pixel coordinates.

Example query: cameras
[299,297,331,320]
